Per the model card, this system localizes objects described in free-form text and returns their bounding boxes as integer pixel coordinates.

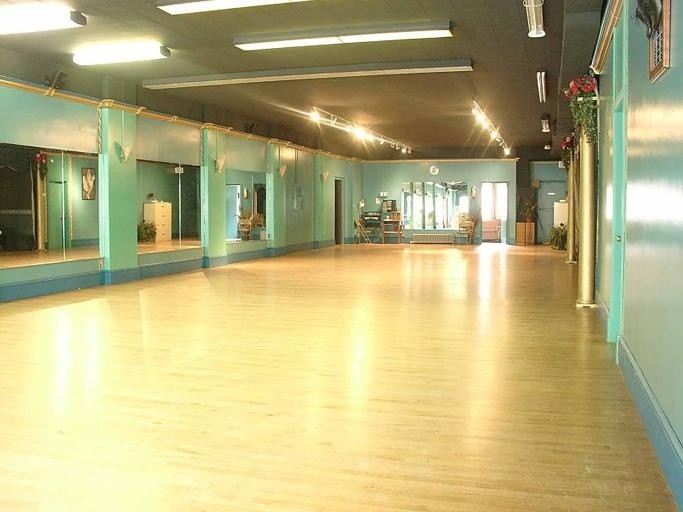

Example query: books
[384,224,394,231]
[393,213,400,219]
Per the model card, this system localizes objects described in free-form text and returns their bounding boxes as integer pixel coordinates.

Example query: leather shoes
[635,0,662,39]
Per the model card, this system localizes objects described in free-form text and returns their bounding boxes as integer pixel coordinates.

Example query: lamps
[230,18,457,52]
[1,7,90,40]
[540,111,553,136]
[138,56,475,97]
[536,64,549,105]
[244,188,248,199]
[157,0,299,18]
[70,38,173,66]
[523,2,549,39]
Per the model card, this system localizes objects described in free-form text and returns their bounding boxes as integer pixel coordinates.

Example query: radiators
[412,233,455,244]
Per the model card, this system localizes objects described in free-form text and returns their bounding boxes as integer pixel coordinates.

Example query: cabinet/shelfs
[459,213,475,243]
[554,201,568,229]
[358,200,403,243]
[142,199,172,241]
[516,222,535,244]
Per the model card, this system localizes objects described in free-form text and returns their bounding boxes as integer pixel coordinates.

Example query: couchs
[482,219,501,242]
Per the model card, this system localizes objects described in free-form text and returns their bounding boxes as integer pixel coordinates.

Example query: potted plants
[138,222,156,242]
[550,226,567,250]
[519,189,537,222]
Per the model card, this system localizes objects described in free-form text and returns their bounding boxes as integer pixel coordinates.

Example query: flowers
[560,70,600,169]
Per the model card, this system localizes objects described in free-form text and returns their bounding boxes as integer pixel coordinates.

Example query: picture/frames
[81,168,97,201]
[644,0,672,86]
